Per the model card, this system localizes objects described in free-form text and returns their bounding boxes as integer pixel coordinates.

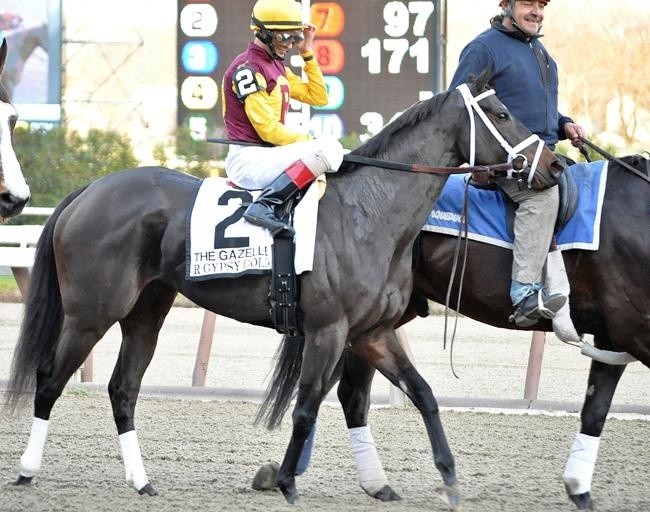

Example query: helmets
[249,0,308,30]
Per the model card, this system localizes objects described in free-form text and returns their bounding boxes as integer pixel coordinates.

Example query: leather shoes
[513,288,567,330]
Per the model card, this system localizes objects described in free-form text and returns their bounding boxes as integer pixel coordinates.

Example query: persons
[447,0,586,332]
[222,0,358,238]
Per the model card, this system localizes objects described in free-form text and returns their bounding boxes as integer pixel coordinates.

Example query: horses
[0,58,565,512]
[252,153,650,512]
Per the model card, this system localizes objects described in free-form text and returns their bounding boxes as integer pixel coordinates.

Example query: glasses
[266,31,306,43]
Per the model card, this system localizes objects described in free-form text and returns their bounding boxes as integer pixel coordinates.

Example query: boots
[242,159,318,239]
